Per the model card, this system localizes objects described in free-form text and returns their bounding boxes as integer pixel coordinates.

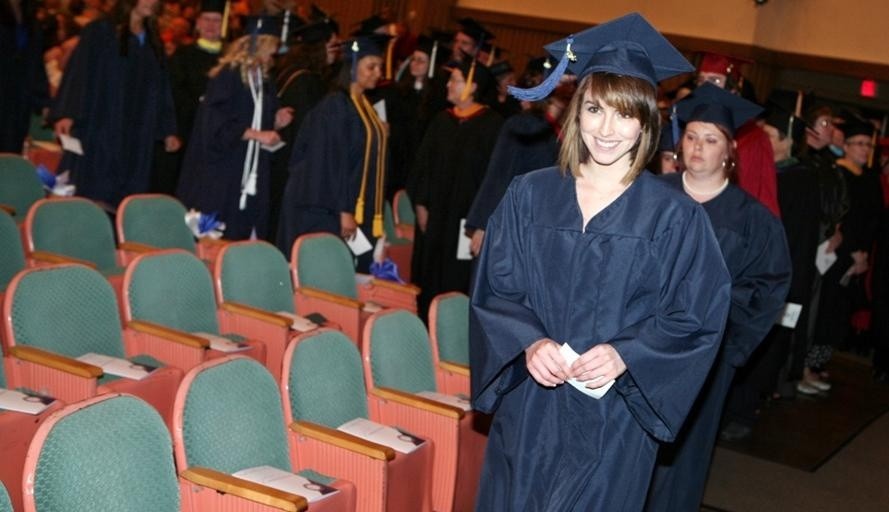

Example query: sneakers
[720,418,753,443]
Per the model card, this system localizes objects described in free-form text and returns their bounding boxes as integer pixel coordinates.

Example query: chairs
[378,189,426,245]
[123,251,296,385]
[292,230,421,348]
[282,328,466,512]
[431,291,471,397]
[213,238,362,355]
[364,310,489,510]
[22,394,300,512]
[120,190,239,254]
[2,265,211,430]
[24,196,158,273]
[0,153,43,218]
[1,210,22,291]
[173,355,393,510]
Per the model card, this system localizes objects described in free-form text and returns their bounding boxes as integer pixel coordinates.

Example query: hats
[540,8,697,92]
[239,2,511,94]
[691,46,889,144]
[199,1,234,38]
[665,81,765,137]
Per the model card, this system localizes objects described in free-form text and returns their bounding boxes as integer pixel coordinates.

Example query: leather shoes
[803,374,833,392]
[792,377,821,395]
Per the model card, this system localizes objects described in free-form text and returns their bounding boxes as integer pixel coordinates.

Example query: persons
[469,12,889,512]
[1,1,576,329]
